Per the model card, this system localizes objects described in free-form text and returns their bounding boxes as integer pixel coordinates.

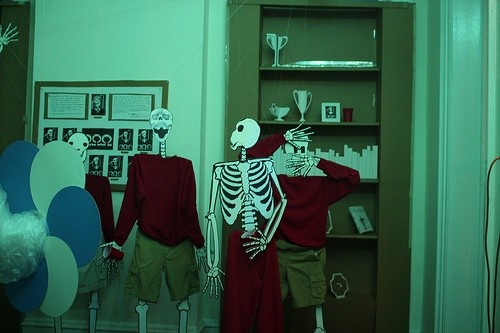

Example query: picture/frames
[322,102,340,122]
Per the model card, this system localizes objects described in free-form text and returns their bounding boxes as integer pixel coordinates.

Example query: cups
[342,107,353,122]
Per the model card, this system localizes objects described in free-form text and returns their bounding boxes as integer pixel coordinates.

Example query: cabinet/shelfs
[220,0,417,333]
